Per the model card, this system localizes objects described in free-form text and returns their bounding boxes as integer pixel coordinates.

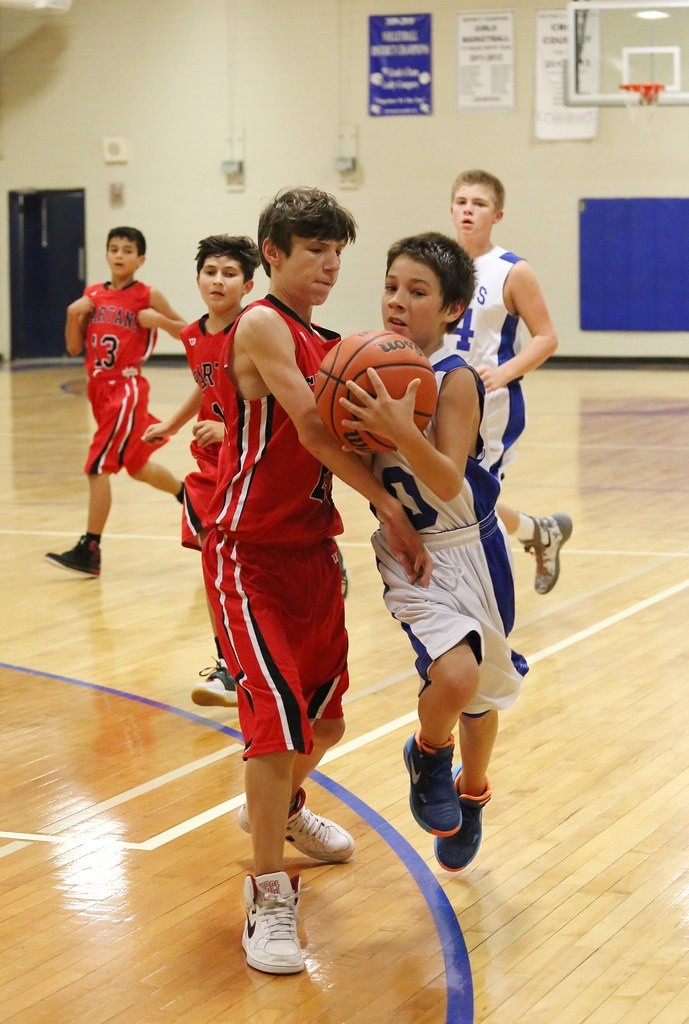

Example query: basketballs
[313,329,438,456]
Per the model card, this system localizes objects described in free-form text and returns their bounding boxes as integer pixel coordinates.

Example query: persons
[140,235,263,709]
[341,232,530,873]
[43,227,191,579]
[442,171,573,593]
[202,187,431,973]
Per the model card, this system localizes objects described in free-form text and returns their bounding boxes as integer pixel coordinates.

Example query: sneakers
[192,656,238,706]
[243,872,304,974]
[237,787,355,861]
[402,729,463,837]
[519,513,573,595]
[43,535,100,577]
[433,763,492,872]
